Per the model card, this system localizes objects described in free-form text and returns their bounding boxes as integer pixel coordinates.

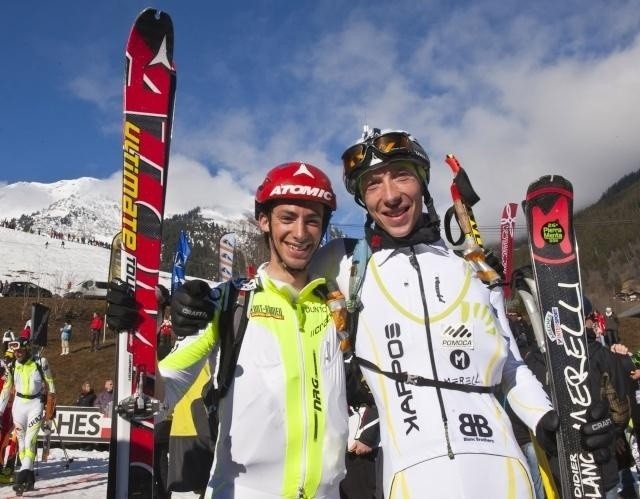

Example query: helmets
[16,337,30,350]
[254,161,337,221]
[341,124,431,197]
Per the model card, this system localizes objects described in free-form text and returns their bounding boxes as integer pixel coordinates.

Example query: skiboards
[108,8,176,499]
[526,174,606,498]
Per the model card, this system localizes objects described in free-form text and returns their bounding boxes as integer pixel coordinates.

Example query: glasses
[9,341,21,351]
[341,132,414,176]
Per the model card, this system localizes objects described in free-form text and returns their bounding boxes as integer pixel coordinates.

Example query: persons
[89,311,103,352]
[106,163,375,497]
[59,320,72,356]
[509,298,638,497]
[76,378,114,407]
[168,125,622,498]
[1,316,57,497]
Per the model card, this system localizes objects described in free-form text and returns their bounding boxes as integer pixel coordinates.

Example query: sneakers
[13,480,34,496]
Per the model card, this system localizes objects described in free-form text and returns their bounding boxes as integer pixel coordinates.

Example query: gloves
[106,290,137,330]
[169,279,215,338]
[534,397,616,464]
